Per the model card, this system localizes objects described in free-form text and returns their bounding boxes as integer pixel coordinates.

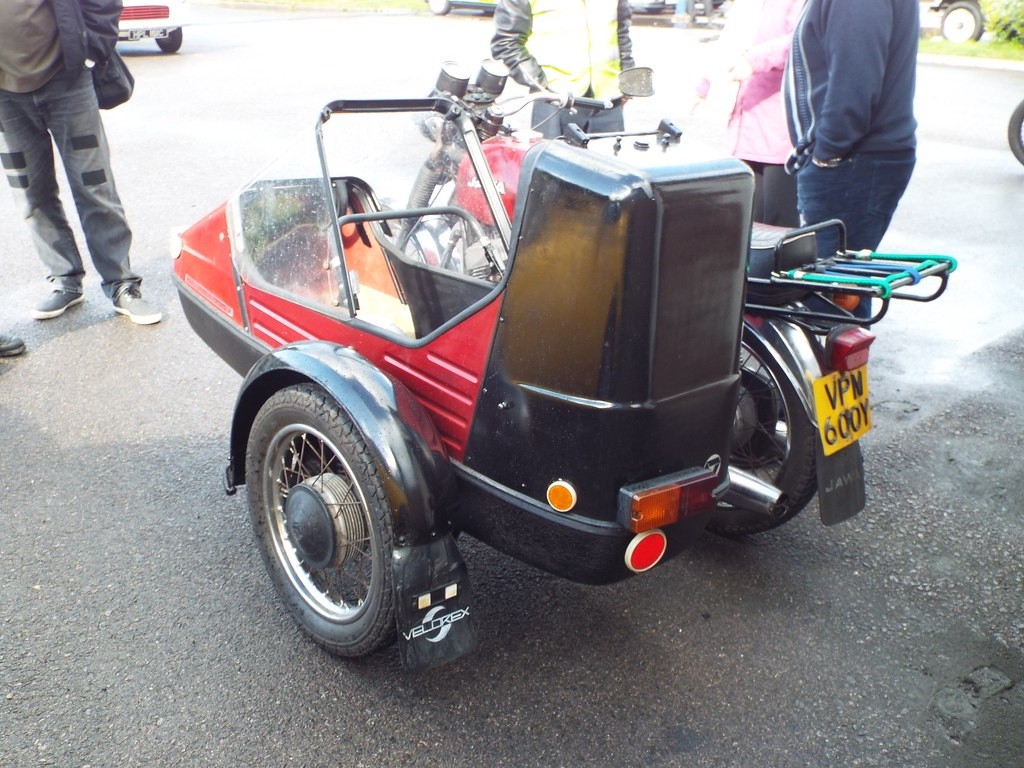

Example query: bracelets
[813,155,841,166]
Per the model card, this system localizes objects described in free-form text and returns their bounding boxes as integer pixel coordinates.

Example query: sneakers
[113,286,162,324]
[31,289,84,319]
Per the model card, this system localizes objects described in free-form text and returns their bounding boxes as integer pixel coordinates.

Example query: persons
[0,334,26,356]
[689,0,812,228]
[490,1,636,140]
[781,0,919,331]
[0,0,162,324]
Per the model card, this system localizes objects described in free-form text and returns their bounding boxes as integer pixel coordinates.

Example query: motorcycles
[168,58,960,685]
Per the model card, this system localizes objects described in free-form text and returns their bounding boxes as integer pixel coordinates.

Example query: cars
[118,1,184,54]
[426,1,726,15]
[926,0,987,43]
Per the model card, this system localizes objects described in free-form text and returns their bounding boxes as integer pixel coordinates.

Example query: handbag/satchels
[92,48,135,110]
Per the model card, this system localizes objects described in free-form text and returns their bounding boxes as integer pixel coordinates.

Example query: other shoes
[0,333,26,356]
